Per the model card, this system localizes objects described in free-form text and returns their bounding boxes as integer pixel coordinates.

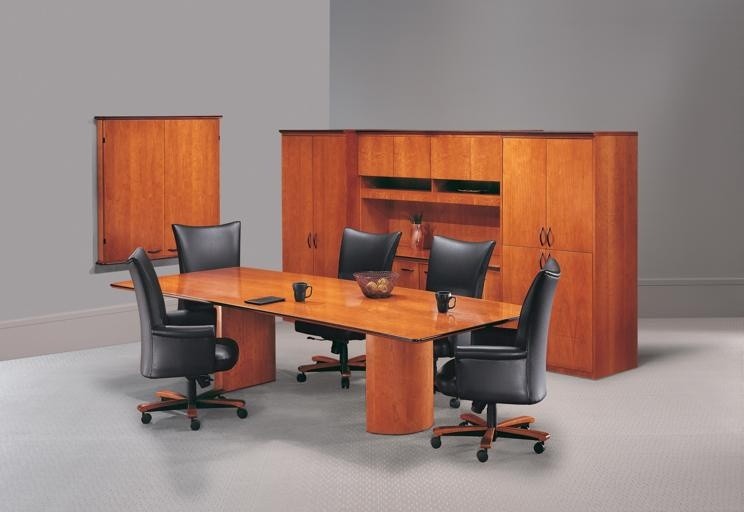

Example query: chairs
[426,235,496,394]
[430,257,561,462]
[296,228,401,388]
[124,221,261,431]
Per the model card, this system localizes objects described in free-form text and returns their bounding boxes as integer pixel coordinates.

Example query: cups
[293,283,312,302]
[435,290,457,313]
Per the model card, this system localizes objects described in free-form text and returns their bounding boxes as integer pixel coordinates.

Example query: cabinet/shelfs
[94,115,221,264]
[504,130,640,380]
[357,129,501,300]
[280,129,356,279]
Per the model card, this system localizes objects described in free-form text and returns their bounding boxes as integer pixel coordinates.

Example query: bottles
[411,224,425,250]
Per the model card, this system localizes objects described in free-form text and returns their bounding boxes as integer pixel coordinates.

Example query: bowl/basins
[353,271,399,298]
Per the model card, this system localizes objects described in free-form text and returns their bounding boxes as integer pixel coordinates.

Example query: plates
[458,189,492,194]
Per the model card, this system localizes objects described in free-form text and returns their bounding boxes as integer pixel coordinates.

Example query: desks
[110,264,523,437]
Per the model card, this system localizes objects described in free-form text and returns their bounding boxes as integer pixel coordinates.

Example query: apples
[366,278,389,293]
